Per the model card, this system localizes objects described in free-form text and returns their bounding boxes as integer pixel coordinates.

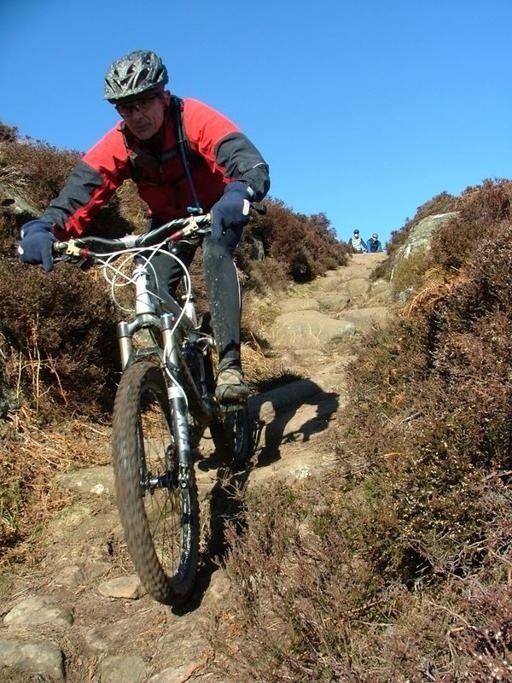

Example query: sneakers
[215,369,249,399]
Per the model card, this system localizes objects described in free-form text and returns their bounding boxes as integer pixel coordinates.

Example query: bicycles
[51,214,251,605]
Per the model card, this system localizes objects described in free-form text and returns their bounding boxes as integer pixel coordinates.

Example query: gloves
[18,220,55,272]
[210,182,257,240]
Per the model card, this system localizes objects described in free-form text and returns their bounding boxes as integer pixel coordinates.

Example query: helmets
[104,50,168,103]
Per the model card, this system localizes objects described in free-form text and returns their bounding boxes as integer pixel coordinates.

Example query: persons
[15,50,270,405]
[367,233,383,253]
[348,229,367,253]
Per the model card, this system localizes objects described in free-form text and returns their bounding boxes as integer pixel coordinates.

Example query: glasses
[115,95,156,117]
